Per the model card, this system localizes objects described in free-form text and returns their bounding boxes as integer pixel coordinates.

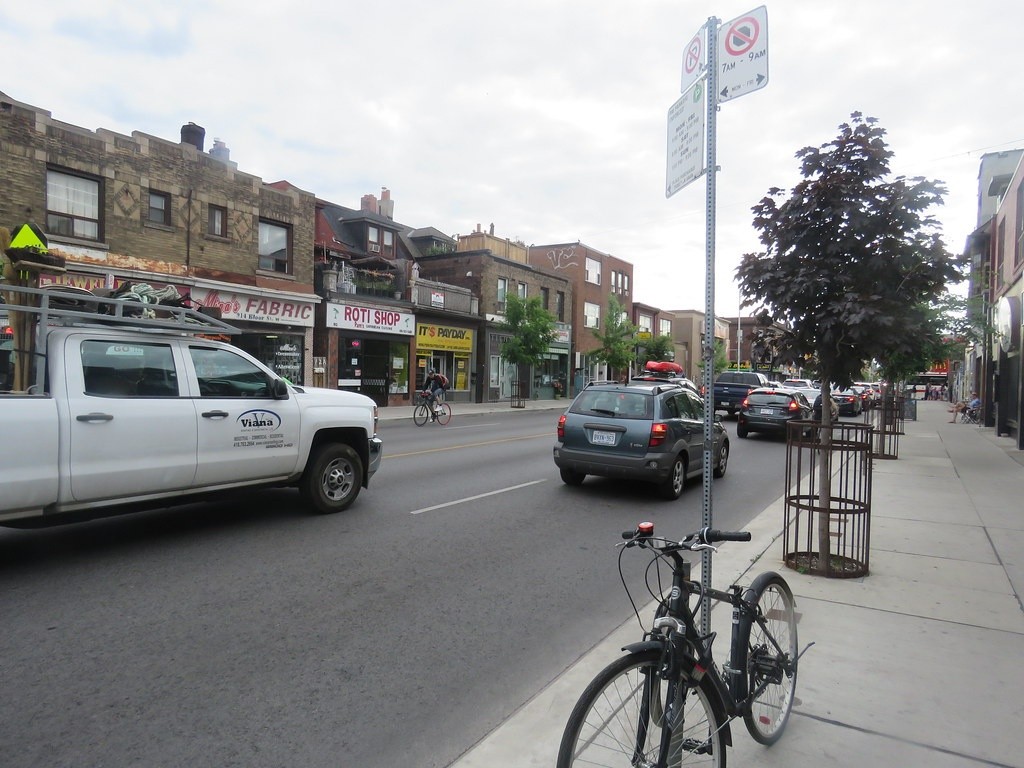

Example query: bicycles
[413,390,451,427]
[555,519,820,768]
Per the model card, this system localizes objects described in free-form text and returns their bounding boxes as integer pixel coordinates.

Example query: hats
[427,370,434,374]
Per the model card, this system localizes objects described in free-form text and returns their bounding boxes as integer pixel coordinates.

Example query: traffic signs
[666,78,705,197]
[715,5,769,103]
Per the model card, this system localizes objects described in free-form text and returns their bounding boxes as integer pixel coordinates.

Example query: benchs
[960,399,980,424]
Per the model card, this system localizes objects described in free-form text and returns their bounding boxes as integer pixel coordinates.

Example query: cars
[737,387,813,440]
[766,377,897,423]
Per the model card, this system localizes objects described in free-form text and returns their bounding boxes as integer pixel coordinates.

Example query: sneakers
[435,405,443,412]
[430,418,434,422]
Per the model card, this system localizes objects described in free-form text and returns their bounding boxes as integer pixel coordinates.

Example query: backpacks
[436,374,450,391]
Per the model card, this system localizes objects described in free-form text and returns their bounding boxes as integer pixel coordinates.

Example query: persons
[422,369,445,423]
[912,382,949,402]
[813,385,836,440]
[947,392,982,423]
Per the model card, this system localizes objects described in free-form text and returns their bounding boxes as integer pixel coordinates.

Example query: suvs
[631,362,701,398]
[553,377,729,500]
[713,372,770,417]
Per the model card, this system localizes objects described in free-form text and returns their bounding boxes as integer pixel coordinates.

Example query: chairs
[137,357,178,398]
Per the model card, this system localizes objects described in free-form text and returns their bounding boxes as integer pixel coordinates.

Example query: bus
[903,371,948,399]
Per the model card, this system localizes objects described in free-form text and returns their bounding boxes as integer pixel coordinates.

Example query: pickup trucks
[1,282,383,533]
